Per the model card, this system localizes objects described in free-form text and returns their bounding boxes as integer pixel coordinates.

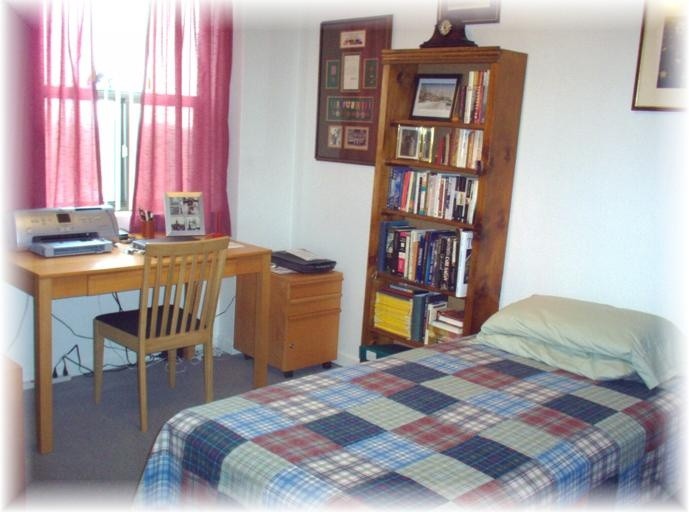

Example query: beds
[158,332,682,509]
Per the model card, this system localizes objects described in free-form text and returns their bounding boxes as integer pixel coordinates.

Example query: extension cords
[23,374,71,390]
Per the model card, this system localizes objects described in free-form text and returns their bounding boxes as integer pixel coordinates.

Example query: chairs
[93,236,229,431]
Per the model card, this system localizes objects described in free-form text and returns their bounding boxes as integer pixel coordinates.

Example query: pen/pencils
[139,208,153,222]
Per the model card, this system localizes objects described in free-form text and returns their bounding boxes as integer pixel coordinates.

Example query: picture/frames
[630,1,685,112]
[395,125,435,163]
[437,1,500,25]
[314,16,393,166]
[163,191,205,237]
[410,74,461,120]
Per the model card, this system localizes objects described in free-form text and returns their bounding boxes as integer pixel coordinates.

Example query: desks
[8,232,271,455]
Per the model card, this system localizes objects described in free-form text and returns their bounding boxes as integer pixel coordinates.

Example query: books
[371,122,483,347]
[452,68,491,127]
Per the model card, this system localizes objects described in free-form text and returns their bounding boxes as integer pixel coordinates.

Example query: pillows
[474,295,680,390]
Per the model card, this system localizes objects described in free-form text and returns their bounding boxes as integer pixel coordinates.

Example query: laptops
[132,236,200,251]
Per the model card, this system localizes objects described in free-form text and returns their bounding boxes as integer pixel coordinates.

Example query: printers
[10,203,122,258]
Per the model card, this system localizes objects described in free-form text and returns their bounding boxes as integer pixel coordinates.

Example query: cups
[141,219,156,240]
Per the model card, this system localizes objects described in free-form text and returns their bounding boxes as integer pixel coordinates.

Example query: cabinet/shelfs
[361,48,527,347]
[233,262,342,378]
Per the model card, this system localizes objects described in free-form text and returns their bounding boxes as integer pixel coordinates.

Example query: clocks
[419,16,478,47]
